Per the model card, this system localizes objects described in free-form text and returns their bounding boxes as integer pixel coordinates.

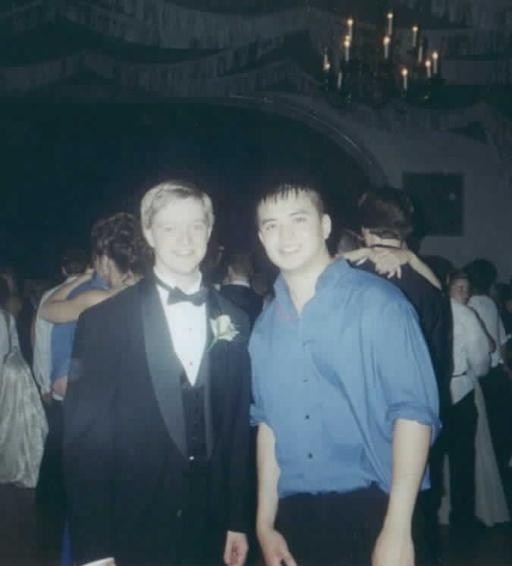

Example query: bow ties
[165,285,213,308]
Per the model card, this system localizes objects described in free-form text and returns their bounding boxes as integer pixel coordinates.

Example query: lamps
[319,0,448,121]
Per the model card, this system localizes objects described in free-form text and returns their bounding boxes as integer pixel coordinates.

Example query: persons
[0,178,512,565]
